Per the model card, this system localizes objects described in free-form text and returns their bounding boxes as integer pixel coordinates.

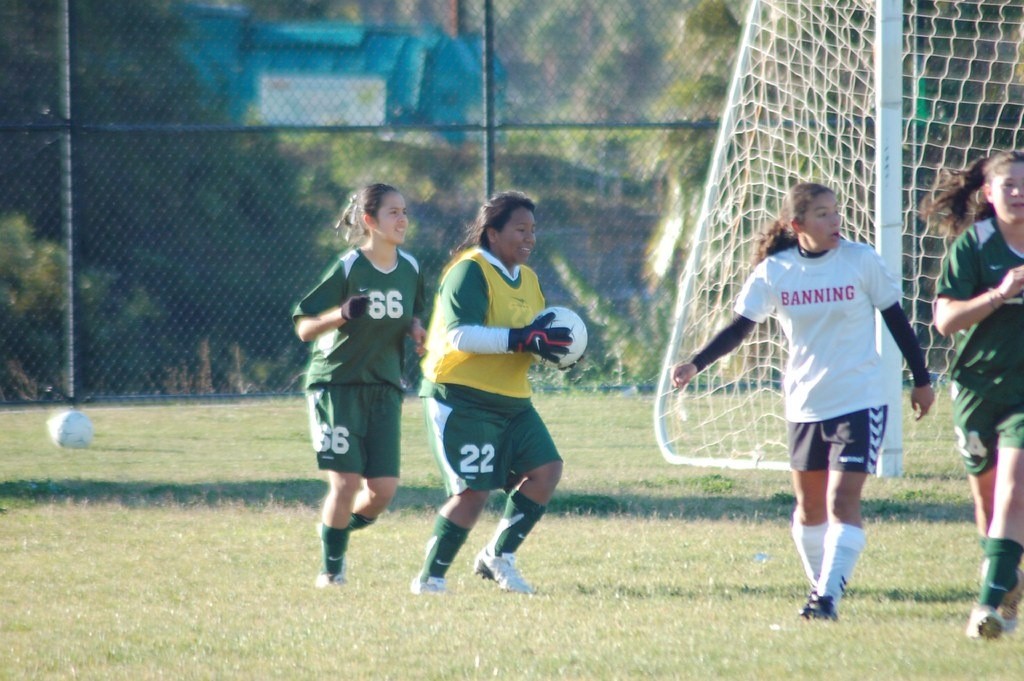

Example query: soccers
[48,411,94,449]
[530,307,587,369]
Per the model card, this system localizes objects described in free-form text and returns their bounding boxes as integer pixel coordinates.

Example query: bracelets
[989,288,1009,310]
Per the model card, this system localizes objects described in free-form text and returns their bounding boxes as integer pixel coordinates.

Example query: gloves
[341,295,369,321]
[557,347,587,371]
[508,312,573,364]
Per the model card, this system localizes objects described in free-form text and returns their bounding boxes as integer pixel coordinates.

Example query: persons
[419,190,586,597]
[919,149,1024,639]
[291,183,428,590]
[669,182,935,624]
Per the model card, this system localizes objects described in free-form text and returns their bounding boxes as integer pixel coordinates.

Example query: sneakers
[410,577,470,611]
[474,547,538,595]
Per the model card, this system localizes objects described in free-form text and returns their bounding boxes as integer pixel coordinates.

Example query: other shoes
[799,589,838,623]
[997,568,1024,633]
[966,602,1004,643]
[315,557,347,590]
